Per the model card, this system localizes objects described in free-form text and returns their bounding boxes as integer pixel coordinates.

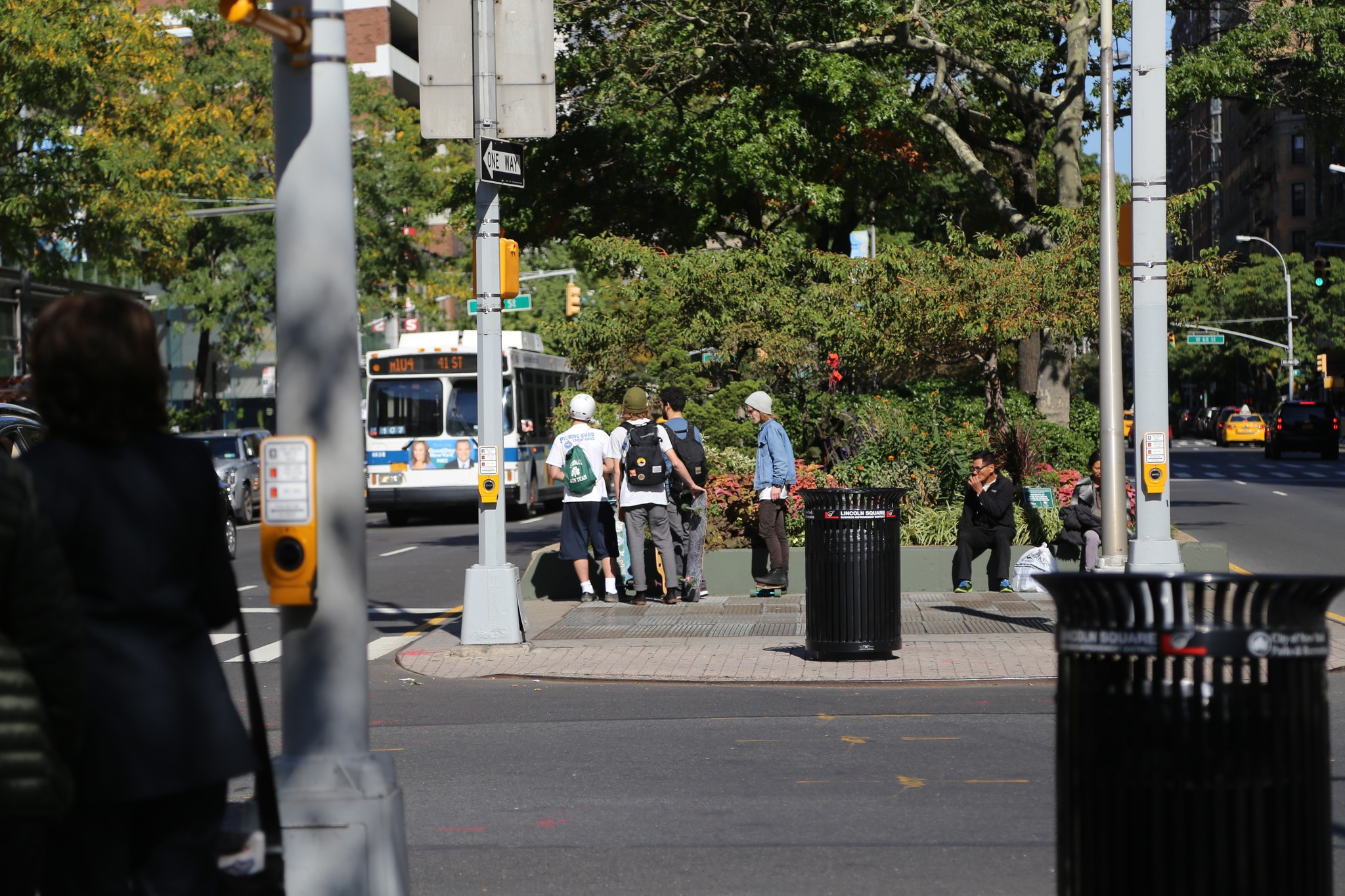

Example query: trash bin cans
[796,488,910,662]
[1031,569,1345,896]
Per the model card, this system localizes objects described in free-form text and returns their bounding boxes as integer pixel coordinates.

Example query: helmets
[568,393,597,422]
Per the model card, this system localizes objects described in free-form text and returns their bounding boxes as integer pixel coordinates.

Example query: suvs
[180,427,272,521]
[1264,400,1340,459]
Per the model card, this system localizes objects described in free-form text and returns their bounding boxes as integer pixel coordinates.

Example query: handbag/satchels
[187,843,287,895]
[1011,541,1059,593]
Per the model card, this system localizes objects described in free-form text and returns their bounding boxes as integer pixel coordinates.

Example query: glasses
[971,463,991,471]
[746,409,754,415]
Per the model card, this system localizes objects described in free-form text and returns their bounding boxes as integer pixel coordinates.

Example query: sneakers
[754,568,789,588]
[999,579,1014,593]
[954,579,973,593]
[629,594,646,606]
[604,588,634,602]
[699,587,709,598]
[662,593,677,604]
[581,588,604,602]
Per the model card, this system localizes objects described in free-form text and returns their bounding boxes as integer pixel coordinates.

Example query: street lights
[1236,235,1299,400]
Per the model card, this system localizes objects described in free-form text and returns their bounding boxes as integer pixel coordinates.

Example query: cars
[1120,401,1265,446]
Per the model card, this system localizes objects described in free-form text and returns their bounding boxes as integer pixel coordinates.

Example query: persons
[742,390,797,590]
[1067,450,1132,573]
[952,451,1016,593]
[0,292,245,896]
[546,386,709,606]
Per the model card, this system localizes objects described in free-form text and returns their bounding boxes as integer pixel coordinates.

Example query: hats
[623,387,648,414]
[744,391,773,415]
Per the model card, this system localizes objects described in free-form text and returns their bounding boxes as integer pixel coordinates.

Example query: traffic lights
[1317,353,1327,378]
[1315,259,1330,290]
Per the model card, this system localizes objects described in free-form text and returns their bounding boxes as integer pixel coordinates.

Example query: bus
[365,329,590,521]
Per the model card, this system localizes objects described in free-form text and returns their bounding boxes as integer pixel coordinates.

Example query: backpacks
[562,428,598,495]
[660,421,709,497]
[618,419,669,486]
